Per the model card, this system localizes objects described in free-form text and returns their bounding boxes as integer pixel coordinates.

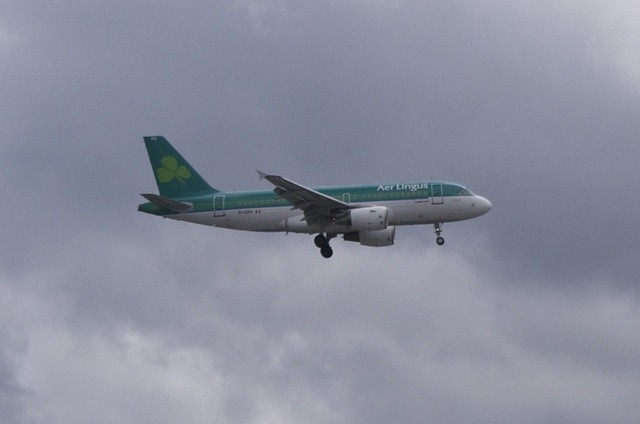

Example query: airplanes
[138,136,492,258]
